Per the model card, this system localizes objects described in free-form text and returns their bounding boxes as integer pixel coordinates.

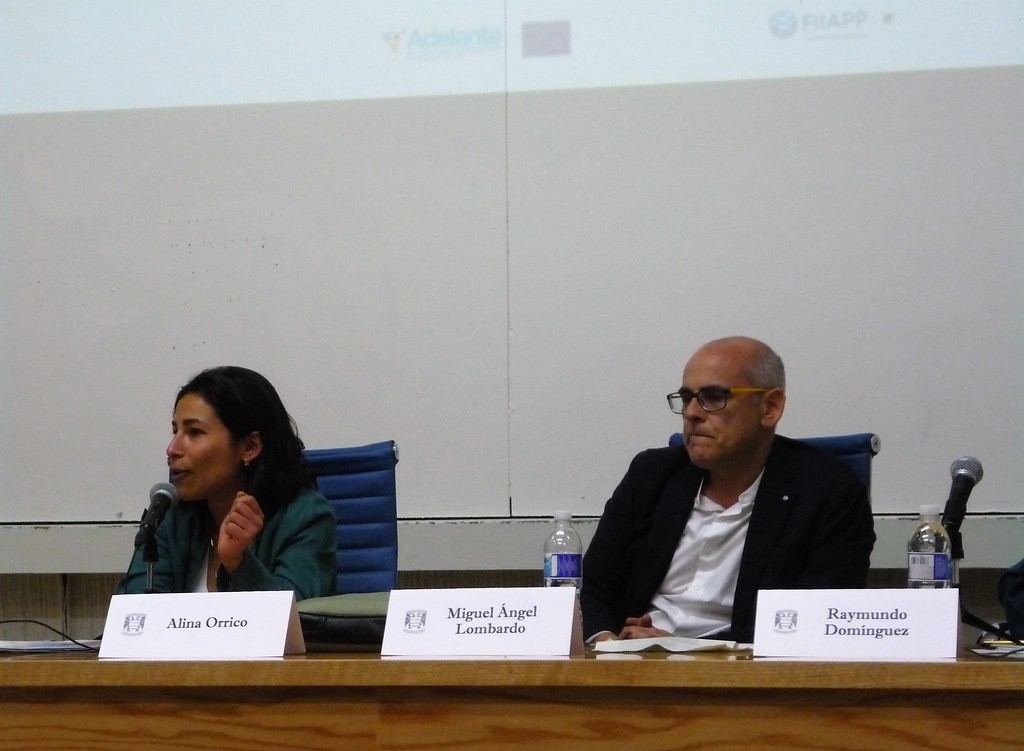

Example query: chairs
[303,440,399,595]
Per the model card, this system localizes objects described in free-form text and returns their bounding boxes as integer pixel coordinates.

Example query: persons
[117,367,337,603]
[580,337,878,644]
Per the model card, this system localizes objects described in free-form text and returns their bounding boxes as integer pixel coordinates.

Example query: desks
[0,649,1024,751]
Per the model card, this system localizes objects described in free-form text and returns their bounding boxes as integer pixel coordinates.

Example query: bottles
[544,511,584,644]
[907,504,951,590]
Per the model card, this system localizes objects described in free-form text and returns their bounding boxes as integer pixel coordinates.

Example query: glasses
[666,387,768,415]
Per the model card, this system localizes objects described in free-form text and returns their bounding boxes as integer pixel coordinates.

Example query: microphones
[941,456,984,541]
[134,483,179,548]
